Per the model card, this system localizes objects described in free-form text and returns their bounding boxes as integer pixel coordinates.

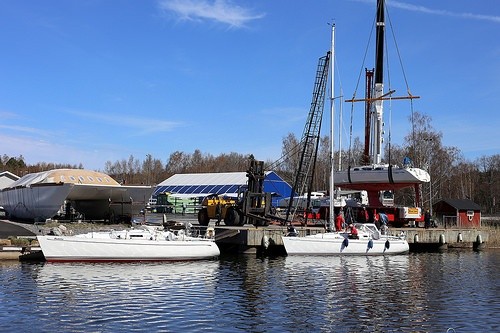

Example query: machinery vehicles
[198,155,291,227]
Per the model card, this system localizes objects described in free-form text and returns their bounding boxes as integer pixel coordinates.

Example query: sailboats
[283,25,411,256]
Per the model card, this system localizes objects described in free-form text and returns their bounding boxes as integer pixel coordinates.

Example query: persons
[402,151,417,169]
[69,204,78,223]
[347,223,360,240]
[214,202,222,226]
[337,206,346,229]
[334,214,344,233]
[360,206,370,223]
[376,211,389,235]
[423,208,433,230]
[285,223,298,237]
[206,228,215,242]
[180,201,188,217]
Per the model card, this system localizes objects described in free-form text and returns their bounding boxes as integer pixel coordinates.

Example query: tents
[148,169,300,206]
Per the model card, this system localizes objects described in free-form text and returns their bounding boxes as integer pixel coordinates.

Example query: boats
[329,161,428,192]
[35,228,219,262]
